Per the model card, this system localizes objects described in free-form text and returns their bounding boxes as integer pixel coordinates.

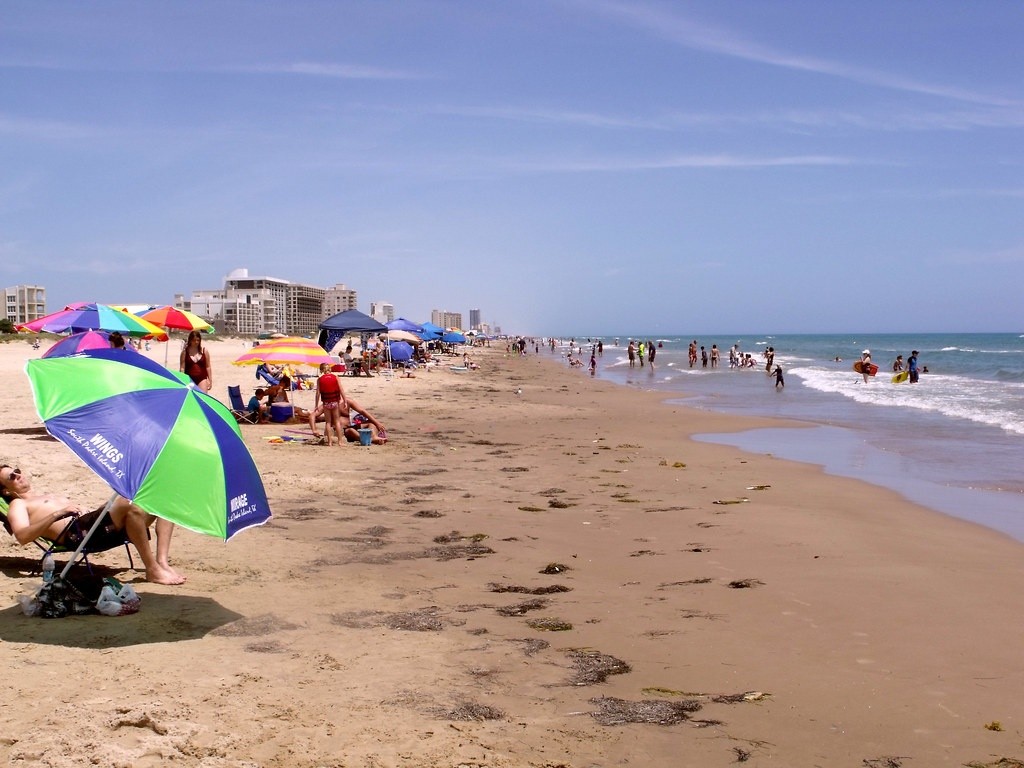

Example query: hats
[912,351,919,354]
[861,349,869,354]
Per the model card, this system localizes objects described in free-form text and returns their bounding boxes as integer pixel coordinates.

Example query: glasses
[5,468,21,485]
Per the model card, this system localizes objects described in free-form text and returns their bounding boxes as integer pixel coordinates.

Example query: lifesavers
[450,366,467,370]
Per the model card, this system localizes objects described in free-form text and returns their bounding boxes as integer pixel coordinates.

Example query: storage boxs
[269,401,293,423]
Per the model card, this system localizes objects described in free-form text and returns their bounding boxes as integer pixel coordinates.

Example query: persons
[0,465,187,585]
[470,338,491,347]
[145,341,150,351]
[129,337,133,345]
[32,336,41,350]
[248,363,389,447]
[463,352,477,369]
[138,339,142,349]
[108,332,125,349]
[179,332,213,395]
[499,335,929,389]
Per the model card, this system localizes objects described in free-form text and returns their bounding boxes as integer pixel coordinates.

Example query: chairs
[0,497,133,576]
[258,370,298,390]
[227,385,259,424]
[342,358,359,377]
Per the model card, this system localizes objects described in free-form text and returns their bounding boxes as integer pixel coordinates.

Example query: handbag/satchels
[96,582,142,616]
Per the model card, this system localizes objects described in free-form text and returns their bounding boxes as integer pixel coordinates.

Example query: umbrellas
[21,302,212,368]
[387,341,414,360]
[378,330,420,354]
[232,336,338,420]
[443,332,466,353]
[24,351,272,583]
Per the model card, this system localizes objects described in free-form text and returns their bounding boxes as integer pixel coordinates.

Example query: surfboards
[853,360,879,376]
[892,372,908,383]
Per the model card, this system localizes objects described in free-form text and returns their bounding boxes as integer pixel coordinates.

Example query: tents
[318,309,444,376]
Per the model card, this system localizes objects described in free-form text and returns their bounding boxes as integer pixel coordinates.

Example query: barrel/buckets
[357,428,372,445]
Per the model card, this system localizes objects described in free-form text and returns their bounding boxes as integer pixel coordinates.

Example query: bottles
[206,377,211,390]
[43,551,54,582]
